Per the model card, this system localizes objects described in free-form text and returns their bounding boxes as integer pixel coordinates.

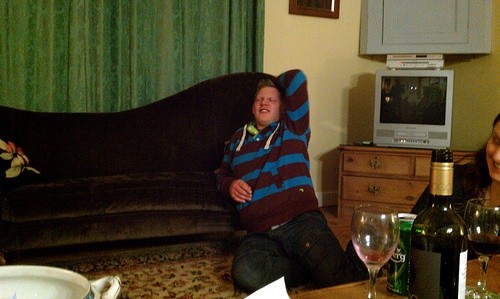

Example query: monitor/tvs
[374,69,453,149]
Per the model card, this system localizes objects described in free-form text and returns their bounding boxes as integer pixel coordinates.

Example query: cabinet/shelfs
[359,0,491,55]
[337,145,477,221]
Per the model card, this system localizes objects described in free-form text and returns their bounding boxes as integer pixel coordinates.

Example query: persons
[214,69,352,294]
[410,113,500,299]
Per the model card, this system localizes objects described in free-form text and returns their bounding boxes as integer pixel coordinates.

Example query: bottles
[408,149,469,299]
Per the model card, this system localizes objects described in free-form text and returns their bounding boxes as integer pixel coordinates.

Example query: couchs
[0,72,278,251]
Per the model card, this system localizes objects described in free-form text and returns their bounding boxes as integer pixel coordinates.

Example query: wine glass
[464,198,500,299]
[350,205,400,299]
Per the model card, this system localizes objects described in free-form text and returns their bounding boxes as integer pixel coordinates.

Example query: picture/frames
[288,0,340,18]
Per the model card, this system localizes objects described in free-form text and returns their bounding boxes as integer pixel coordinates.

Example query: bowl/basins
[0,266,121,299]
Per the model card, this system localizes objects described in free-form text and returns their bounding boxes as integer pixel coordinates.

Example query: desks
[289,254,500,299]
